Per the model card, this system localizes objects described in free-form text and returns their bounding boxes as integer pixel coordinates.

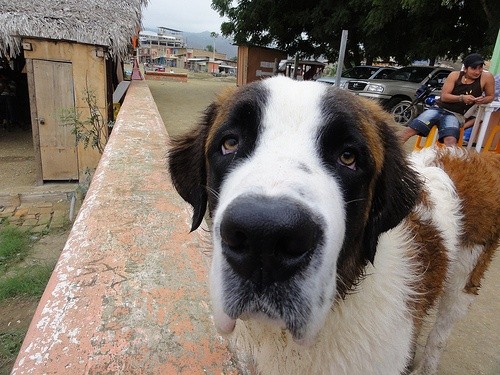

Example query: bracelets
[460,94,464,103]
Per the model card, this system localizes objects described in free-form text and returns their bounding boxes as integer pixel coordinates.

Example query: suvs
[344,65,460,125]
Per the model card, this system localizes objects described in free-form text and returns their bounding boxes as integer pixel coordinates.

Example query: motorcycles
[390,73,477,145]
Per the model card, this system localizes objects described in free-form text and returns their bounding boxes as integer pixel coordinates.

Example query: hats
[464,54,486,67]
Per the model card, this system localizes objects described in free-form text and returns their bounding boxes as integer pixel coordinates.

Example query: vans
[274,59,326,82]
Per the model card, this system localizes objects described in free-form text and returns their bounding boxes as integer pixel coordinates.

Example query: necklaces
[464,74,467,84]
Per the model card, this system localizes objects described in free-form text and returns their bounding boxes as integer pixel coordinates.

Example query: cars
[316,66,410,86]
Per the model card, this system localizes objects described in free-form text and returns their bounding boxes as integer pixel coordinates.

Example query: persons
[399,54,495,148]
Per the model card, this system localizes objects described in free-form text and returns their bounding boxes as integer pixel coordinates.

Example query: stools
[482,126,500,154]
[413,125,464,152]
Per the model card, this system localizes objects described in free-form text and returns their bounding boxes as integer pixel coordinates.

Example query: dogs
[165,75,500,375]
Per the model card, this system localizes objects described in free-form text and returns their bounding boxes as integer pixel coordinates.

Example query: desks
[467,101,500,153]
